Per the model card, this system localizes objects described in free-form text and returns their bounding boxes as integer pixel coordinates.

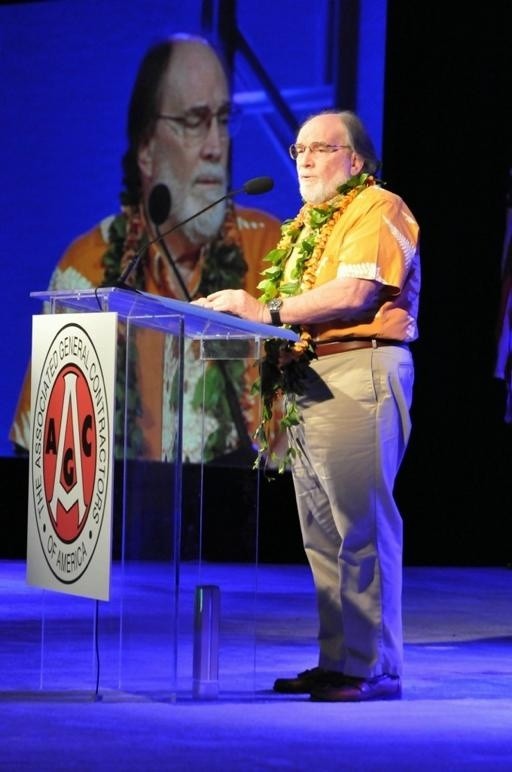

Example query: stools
[305,337,407,358]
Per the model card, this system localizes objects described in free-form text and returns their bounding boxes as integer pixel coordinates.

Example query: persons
[6,29,291,471]
[185,108,424,703]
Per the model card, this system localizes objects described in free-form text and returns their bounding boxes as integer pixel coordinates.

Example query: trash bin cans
[268,297,285,326]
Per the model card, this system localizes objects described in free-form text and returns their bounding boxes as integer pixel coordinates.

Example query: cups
[289,143,355,160]
[150,101,240,140]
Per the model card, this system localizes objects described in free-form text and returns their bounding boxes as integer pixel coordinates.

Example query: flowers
[146,183,270,471]
[102,172,277,295]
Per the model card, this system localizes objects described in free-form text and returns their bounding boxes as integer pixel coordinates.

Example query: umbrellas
[273,666,402,702]
